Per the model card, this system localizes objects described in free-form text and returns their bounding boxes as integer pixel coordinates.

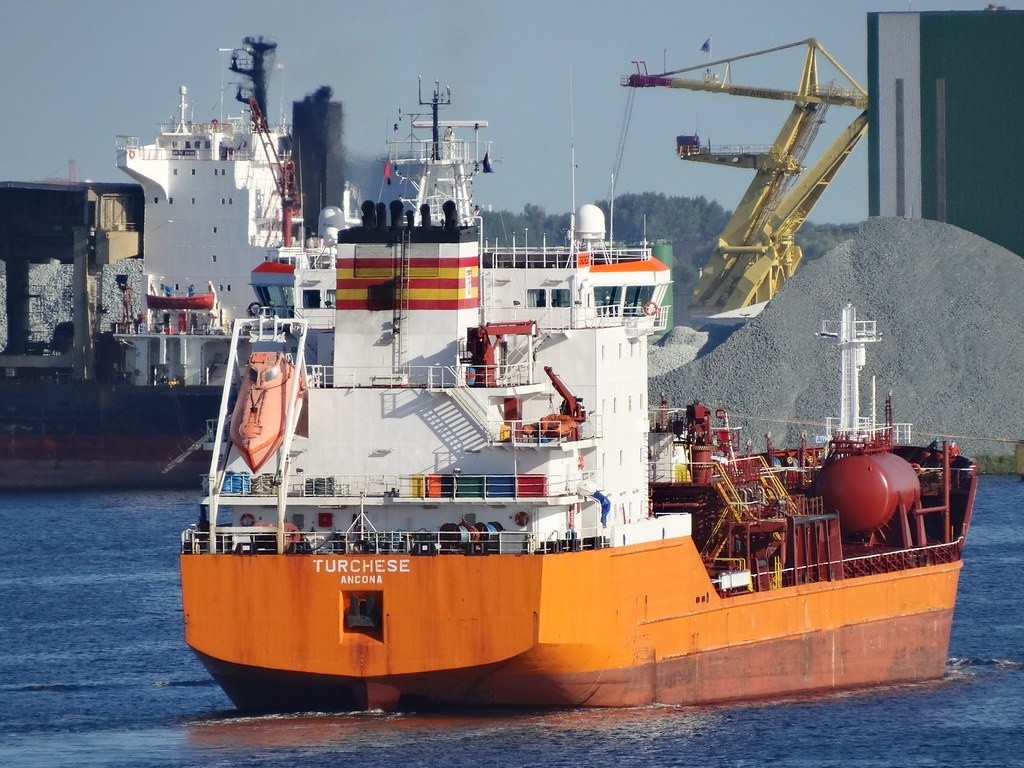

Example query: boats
[155,71,979,714]
[228,350,300,477]
[145,291,216,311]
[539,413,577,438]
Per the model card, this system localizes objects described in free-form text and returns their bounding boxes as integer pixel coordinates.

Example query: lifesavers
[644,301,657,316]
[240,513,256,526]
[515,511,529,526]
[248,302,262,316]
